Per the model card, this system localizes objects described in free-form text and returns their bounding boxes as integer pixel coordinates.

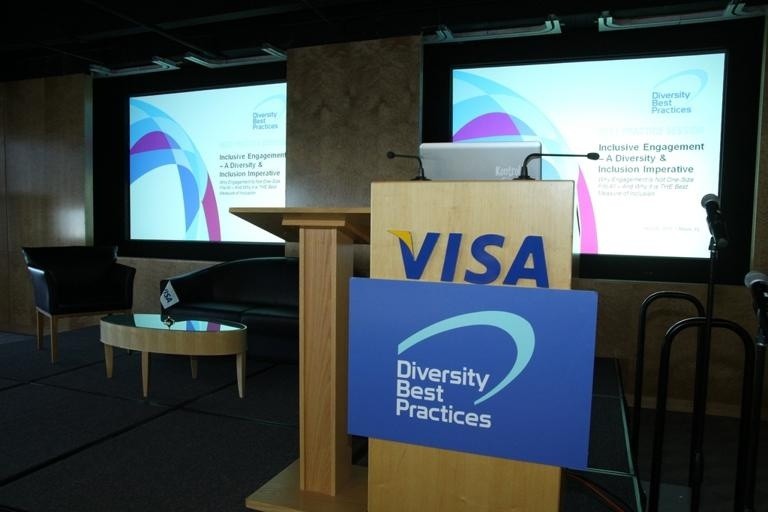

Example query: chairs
[19,244,138,365]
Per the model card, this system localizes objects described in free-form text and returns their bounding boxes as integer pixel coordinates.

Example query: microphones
[515,153,599,180]
[745,271,768,338]
[387,152,429,180]
[701,194,727,248]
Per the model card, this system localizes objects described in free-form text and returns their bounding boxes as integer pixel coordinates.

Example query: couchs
[159,255,299,367]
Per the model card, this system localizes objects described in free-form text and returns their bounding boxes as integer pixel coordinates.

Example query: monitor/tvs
[419,142,542,180]
